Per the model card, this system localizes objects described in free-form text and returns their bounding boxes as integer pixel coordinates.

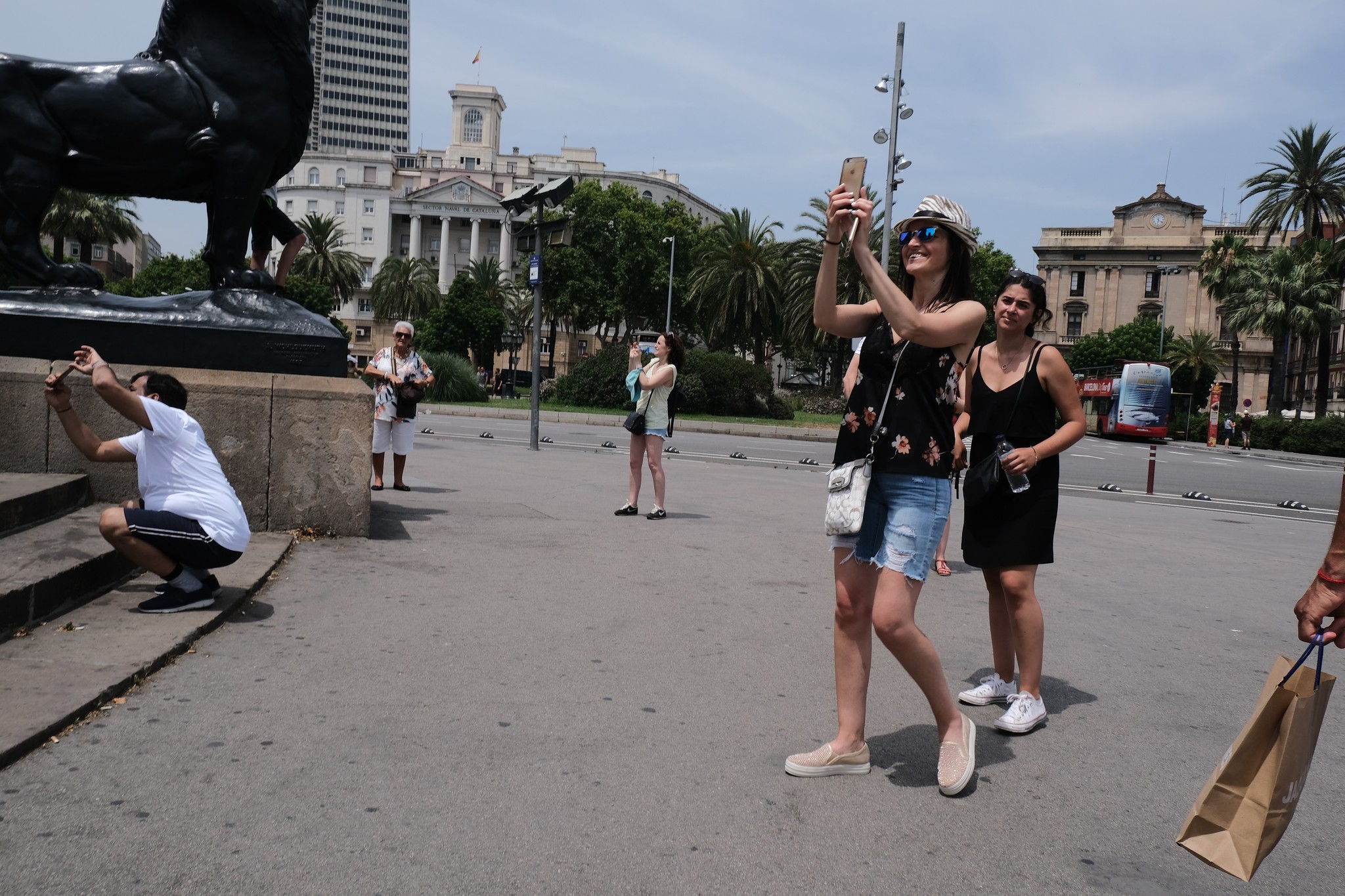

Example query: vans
[501,368,549,388]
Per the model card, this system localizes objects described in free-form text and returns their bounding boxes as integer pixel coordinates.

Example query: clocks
[1151,212,1166,227]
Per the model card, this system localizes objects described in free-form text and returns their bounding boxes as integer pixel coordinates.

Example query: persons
[474,366,504,400]
[1224,414,1236,449]
[784,186,988,797]
[347,360,361,379]
[614,331,686,519]
[249,183,307,297]
[1318,568,1345,586]
[364,320,436,491]
[1241,410,1253,451]
[843,335,866,404]
[933,367,966,576]
[1294,451,1345,650]
[951,270,1088,734]
[45,345,251,614]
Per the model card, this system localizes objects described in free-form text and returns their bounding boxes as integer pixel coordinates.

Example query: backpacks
[667,379,686,437]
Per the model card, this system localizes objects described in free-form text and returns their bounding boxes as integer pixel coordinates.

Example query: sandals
[935,560,951,576]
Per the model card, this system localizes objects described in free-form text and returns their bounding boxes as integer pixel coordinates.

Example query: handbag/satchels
[825,458,873,535]
[623,412,644,436]
[396,381,424,419]
[1174,628,1336,880]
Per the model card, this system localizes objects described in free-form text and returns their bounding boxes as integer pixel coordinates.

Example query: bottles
[996,434,1031,493]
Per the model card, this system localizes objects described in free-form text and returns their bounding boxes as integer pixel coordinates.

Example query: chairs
[1094,403,1110,414]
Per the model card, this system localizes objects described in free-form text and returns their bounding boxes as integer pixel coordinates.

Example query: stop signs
[582,352,589,358]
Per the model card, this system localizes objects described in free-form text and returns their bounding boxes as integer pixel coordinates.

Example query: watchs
[92,360,111,369]
[425,380,429,388]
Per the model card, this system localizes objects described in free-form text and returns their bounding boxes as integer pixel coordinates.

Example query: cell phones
[838,157,868,210]
[635,335,640,349]
[50,362,80,387]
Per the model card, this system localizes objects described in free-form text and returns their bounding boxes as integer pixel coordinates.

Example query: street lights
[1157,265,1182,361]
[499,331,523,393]
[872,21,916,273]
[777,364,783,389]
[661,236,676,334]
[498,174,576,453]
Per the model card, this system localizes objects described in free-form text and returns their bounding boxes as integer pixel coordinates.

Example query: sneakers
[958,673,1015,705]
[647,504,666,520]
[994,689,1046,732]
[137,574,222,612]
[614,498,638,515]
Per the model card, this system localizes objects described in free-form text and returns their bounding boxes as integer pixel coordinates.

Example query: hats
[626,367,643,403]
[892,195,978,258]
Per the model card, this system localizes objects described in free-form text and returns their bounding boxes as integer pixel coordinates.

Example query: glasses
[899,224,959,248]
[1008,269,1048,294]
[395,332,411,339]
[667,331,675,343]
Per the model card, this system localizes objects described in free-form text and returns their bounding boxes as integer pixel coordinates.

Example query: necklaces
[994,338,1030,370]
[394,345,408,360]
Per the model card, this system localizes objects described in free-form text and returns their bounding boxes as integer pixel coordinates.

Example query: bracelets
[383,371,388,380]
[951,395,957,407]
[824,236,842,245]
[56,406,72,413]
[1030,445,1038,466]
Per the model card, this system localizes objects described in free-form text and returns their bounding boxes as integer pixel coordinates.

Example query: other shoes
[784,740,870,778]
[936,710,976,796]
[393,482,410,491]
[371,482,384,490]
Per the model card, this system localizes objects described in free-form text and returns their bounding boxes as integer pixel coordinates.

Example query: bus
[1073,358,1173,439]
